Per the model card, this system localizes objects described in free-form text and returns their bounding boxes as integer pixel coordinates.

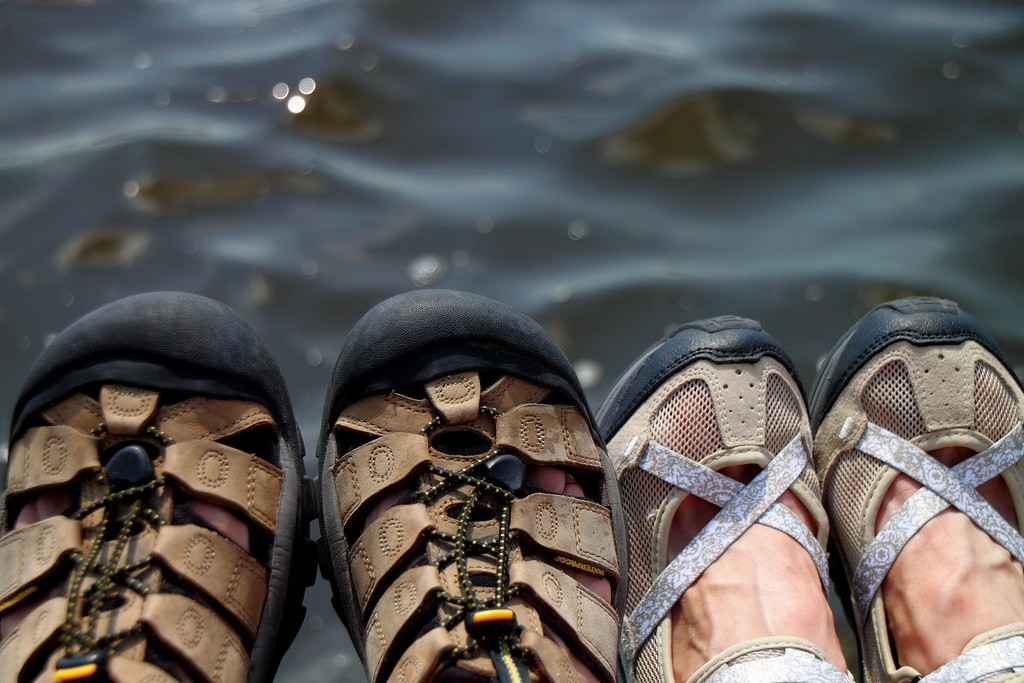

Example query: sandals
[0,291,318,683]
[313,289,631,683]
[594,315,854,683]
[808,296,1024,683]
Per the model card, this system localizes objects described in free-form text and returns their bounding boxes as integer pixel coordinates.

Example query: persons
[1,287,630,682]
[592,294,1023,682]
